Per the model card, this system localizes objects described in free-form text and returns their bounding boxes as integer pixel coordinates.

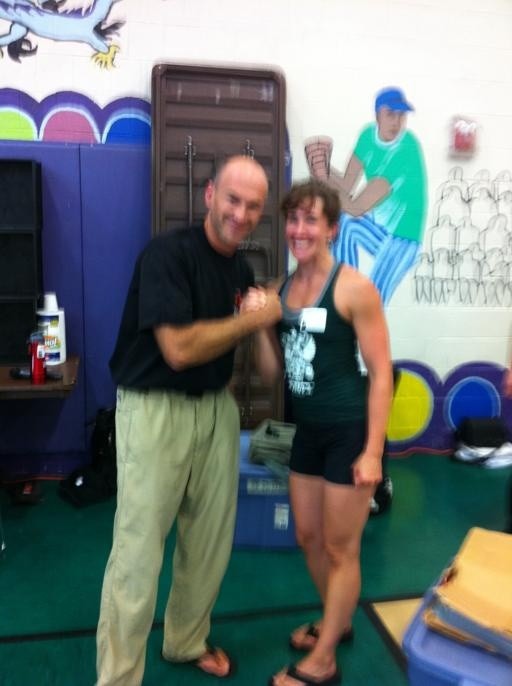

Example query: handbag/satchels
[459,416,509,447]
[249,417,297,466]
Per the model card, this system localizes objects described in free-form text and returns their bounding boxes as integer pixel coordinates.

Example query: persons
[98,156,282,685]
[240,180,392,686]
[304,87,427,313]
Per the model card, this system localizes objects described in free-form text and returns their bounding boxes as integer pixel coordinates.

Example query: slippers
[290,620,353,651]
[269,662,341,685]
[188,643,236,678]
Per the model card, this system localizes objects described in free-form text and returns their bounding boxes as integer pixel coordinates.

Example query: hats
[375,86,415,113]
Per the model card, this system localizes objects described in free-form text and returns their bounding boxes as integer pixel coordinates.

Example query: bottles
[37,293,68,366]
